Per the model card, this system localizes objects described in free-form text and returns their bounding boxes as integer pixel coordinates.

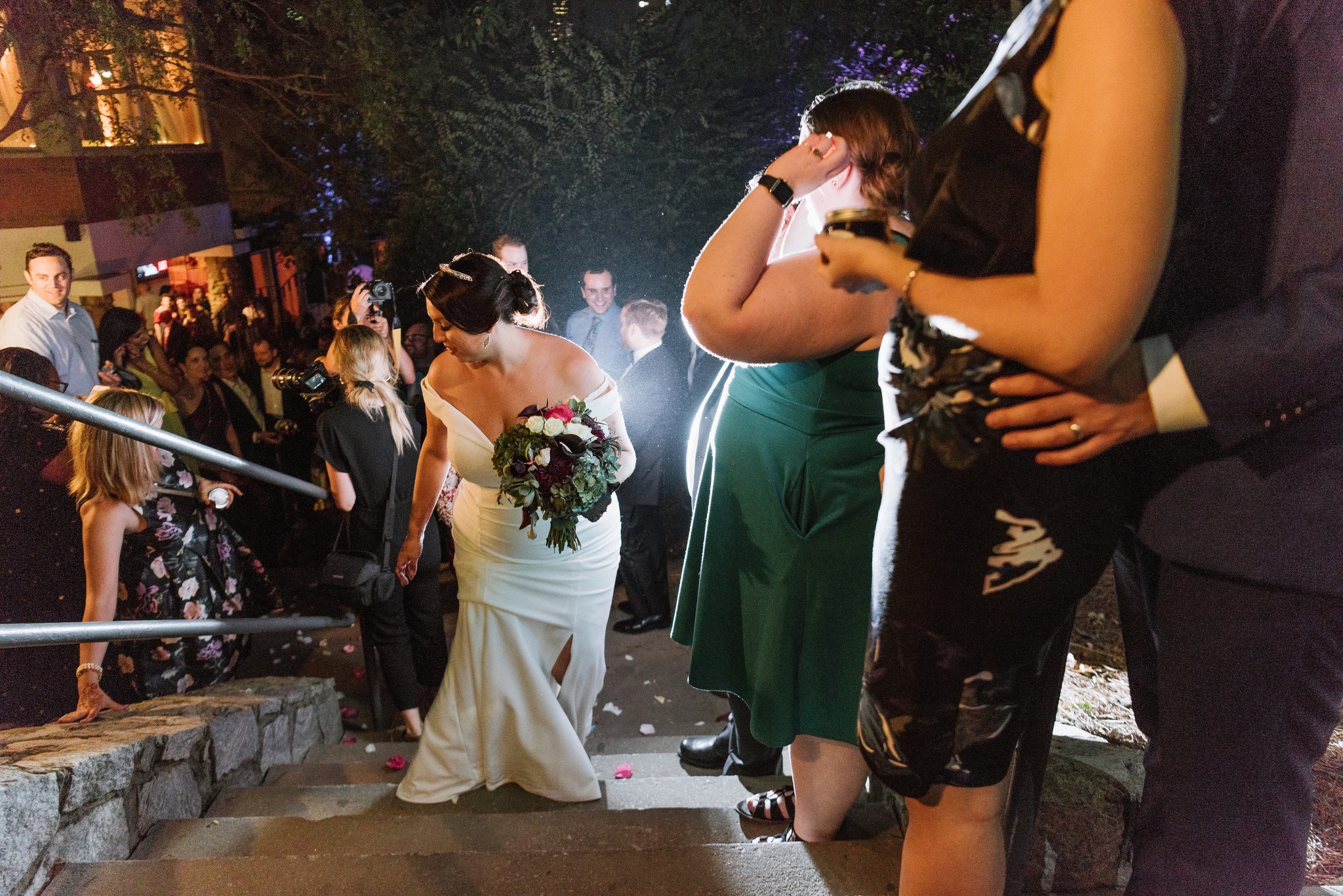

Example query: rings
[803,142,812,150]
[812,149,823,159]
[1069,421,1081,441]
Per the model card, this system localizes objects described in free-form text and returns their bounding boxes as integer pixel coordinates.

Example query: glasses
[44,378,69,393]
[405,333,431,341]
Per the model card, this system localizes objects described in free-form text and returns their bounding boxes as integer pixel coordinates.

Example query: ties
[583,317,600,352]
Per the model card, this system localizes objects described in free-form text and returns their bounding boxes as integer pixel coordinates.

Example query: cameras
[271,360,332,396]
[361,281,391,302]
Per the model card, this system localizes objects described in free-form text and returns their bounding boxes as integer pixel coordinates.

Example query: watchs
[758,175,793,207]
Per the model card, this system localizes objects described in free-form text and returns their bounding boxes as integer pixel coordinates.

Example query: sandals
[736,784,796,822]
[745,825,803,844]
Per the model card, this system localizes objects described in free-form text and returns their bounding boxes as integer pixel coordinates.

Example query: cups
[820,208,891,287]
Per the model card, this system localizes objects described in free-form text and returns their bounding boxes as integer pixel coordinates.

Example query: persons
[815,0,1217,896]
[567,260,684,634]
[668,84,920,844]
[0,242,102,398]
[395,251,636,804]
[492,233,528,274]
[0,279,460,742]
[984,0,1343,896]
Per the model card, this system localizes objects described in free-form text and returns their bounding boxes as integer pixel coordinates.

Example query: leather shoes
[676,736,726,768]
[619,600,634,612]
[612,612,673,633]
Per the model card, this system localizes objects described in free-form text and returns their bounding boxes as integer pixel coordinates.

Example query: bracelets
[904,265,922,319]
[76,663,103,680]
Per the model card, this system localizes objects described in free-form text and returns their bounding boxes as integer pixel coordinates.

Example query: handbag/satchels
[321,552,394,606]
